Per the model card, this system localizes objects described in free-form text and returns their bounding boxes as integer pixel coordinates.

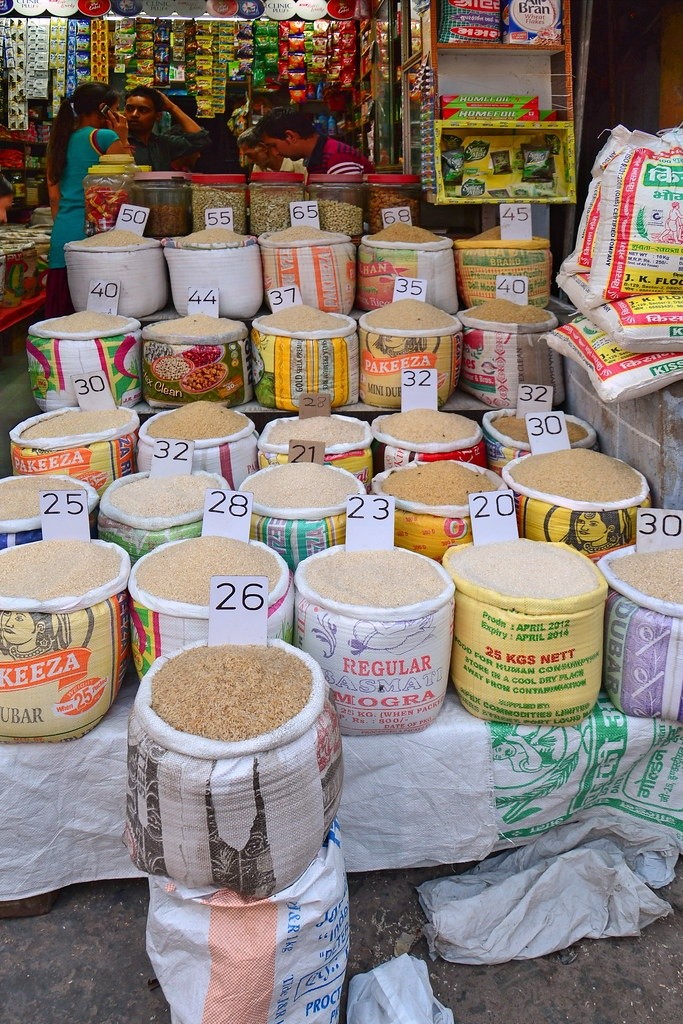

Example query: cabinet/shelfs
[421,0,578,206]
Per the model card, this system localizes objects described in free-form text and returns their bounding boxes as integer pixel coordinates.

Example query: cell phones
[100,105,119,121]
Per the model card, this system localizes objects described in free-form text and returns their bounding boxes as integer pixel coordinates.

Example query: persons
[45,83,136,321]
[125,86,210,172]
[163,125,200,172]
[0,175,14,224]
[254,107,377,175]
[237,126,309,184]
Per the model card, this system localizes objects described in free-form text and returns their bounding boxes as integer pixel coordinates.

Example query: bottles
[329,115,337,136]
[319,112,328,135]
[308,81,316,100]
[317,81,323,99]
[11,172,47,209]
[83,155,420,239]
